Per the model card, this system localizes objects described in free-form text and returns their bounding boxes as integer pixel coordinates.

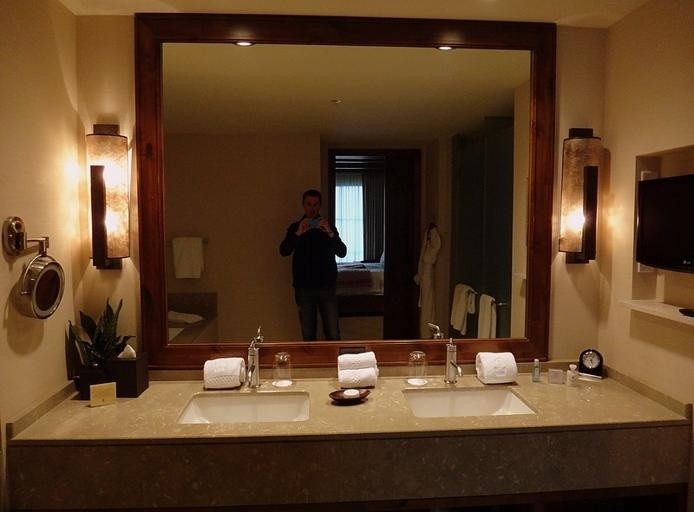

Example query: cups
[408,351,428,384]
[273,352,292,387]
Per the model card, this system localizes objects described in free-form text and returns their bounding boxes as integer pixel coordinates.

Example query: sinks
[401,384,539,418]
[174,390,312,425]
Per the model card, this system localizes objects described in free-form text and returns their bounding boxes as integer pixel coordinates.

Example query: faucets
[427,322,443,340]
[254,326,265,343]
[440,338,466,383]
[244,339,264,390]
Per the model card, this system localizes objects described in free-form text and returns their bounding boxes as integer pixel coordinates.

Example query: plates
[329,388,370,403]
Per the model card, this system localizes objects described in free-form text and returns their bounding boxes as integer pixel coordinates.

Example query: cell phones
[309,219,320,226]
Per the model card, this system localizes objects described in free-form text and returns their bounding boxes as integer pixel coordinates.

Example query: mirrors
[130,11,554,371]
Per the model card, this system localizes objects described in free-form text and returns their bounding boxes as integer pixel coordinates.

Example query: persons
[279,188,348,343]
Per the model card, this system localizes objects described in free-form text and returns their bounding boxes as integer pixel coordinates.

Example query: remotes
[679,308,694,317]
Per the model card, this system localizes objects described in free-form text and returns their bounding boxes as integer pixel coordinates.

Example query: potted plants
[62,292,150,399]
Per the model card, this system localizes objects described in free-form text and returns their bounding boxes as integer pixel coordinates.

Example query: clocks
[579,349,603,376]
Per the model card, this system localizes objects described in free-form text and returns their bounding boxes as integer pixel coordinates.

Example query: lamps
[83,122,131,271]
[558,127,603,265]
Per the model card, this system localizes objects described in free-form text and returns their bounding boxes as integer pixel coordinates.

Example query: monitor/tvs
[635,172,694,275]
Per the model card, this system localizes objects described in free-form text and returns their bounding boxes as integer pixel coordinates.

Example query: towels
[204,357,246,391]
[172,236,205,279]
[474,351,518,385]
[449,283,500,339]
[337,350,379,390]
